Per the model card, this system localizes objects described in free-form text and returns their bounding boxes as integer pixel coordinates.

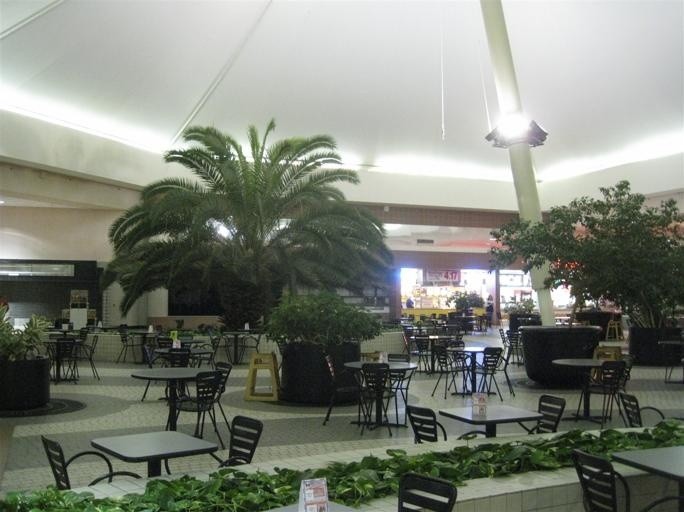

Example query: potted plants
[263,285,384,404]
[0,303,57,410]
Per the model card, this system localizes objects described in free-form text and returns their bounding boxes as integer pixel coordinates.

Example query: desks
[264,501,357,512]
[611,445,684,512]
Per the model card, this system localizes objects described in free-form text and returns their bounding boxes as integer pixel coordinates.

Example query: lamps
[487,116,547,150]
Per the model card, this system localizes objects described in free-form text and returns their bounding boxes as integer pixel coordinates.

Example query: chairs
[398,474,457,512]
[569,447,684,512]
[39,320,263,491]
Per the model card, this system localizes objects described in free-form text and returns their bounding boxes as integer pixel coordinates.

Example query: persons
[485,294,493,329]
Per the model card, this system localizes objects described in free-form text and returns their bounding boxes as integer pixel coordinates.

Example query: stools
[242,352,280,402]
